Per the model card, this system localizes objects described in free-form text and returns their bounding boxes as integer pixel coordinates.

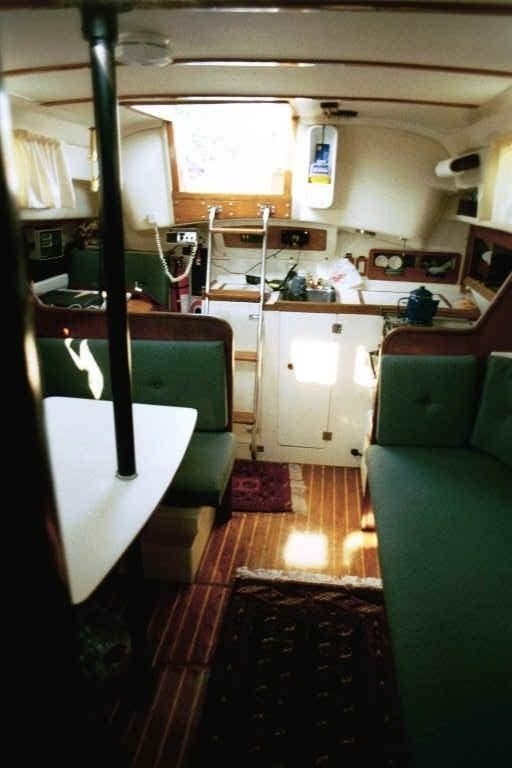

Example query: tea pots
[398,285,440,327]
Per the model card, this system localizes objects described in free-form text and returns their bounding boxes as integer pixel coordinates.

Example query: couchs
[61,247,171,311]
[362,272,512,768]
[31,305,238,584]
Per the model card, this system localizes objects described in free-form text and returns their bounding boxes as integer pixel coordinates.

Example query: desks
[41,397,198,604]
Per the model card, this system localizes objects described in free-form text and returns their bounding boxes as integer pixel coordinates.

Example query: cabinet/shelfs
[251,311,384,467]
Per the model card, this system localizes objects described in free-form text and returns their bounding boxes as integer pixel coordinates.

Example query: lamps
[114,31,174,70]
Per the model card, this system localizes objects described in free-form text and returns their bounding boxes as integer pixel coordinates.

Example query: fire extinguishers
[172,256,192,312]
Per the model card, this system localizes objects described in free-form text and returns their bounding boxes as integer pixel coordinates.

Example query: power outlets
[280,229,310,246]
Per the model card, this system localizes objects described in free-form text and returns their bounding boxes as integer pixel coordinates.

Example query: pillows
[470,350,511,470]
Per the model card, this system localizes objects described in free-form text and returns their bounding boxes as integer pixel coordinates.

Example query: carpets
[182,566,410,768]
[231,458,308,513]
